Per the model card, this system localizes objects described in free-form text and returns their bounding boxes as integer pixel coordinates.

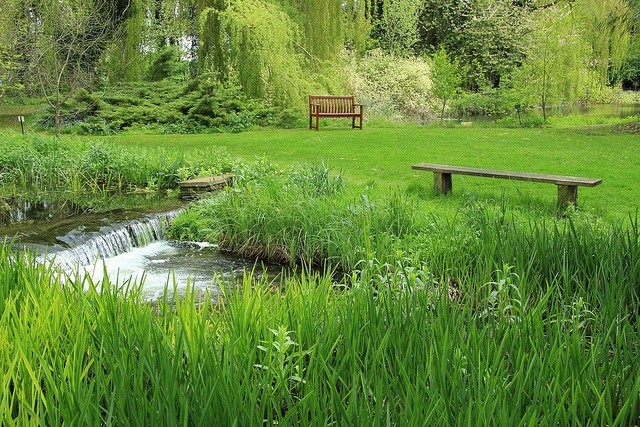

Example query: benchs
[307,94,364,132]
[411,160,602,218]
